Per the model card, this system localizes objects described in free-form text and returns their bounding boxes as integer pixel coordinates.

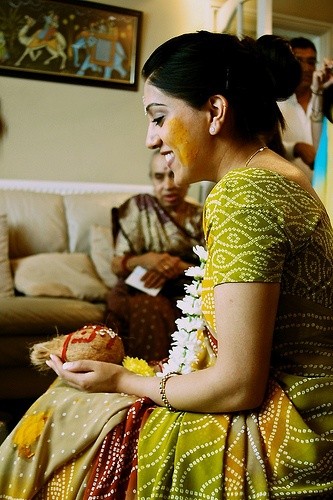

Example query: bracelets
[311,116,321,122]
[311,108,321,114]
[311,86,323,96]
[160,373,181,412]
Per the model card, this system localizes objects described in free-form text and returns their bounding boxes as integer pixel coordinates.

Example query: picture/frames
[0,0,143,91]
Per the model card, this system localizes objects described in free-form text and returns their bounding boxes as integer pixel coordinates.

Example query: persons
[105,149,206,364]
[0,29,333,500]
[310,56,333,231]
[274,36,317,184]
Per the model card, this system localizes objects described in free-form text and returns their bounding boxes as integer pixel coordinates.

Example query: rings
[165,266,170,270]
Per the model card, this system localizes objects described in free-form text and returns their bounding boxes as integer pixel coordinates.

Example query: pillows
[12,252,107,301]
[88,221,119,287]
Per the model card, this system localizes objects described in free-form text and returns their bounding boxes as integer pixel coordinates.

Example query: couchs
[0,189,199,365]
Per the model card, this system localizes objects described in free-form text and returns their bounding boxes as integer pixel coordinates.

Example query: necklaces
[245,147,268,167]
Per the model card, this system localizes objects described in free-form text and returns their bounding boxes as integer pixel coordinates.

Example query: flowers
[122,245,210,377]
